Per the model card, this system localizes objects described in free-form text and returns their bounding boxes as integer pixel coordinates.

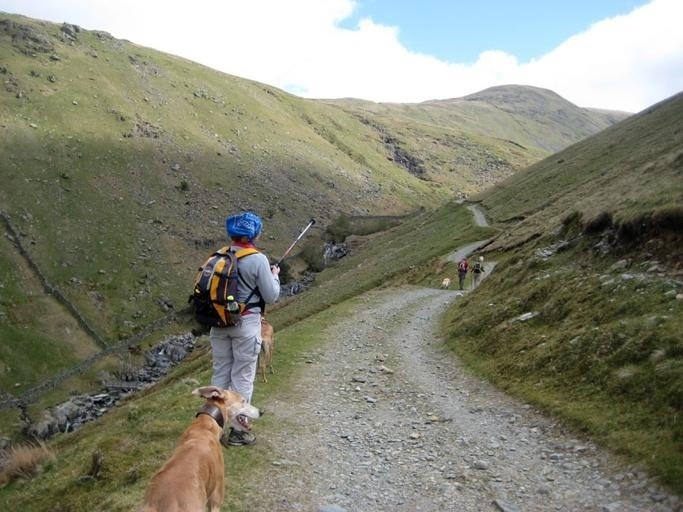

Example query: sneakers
[227,431,255,446]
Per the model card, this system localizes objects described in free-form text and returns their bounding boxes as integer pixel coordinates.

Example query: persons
[457,258,469,290]
[209,214,280,447]
[470,256,486,289]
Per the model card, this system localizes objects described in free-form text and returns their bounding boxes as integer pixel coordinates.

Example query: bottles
[227,295,242,327]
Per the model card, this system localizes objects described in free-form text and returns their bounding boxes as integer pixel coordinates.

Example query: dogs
[257,316,276,375]
[139,384,266,512]
[442,278,451,289]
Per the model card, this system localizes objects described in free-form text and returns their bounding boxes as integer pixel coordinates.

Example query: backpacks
[193,247,259,327]
[473,263,482,273]
[458,262,465,271]
